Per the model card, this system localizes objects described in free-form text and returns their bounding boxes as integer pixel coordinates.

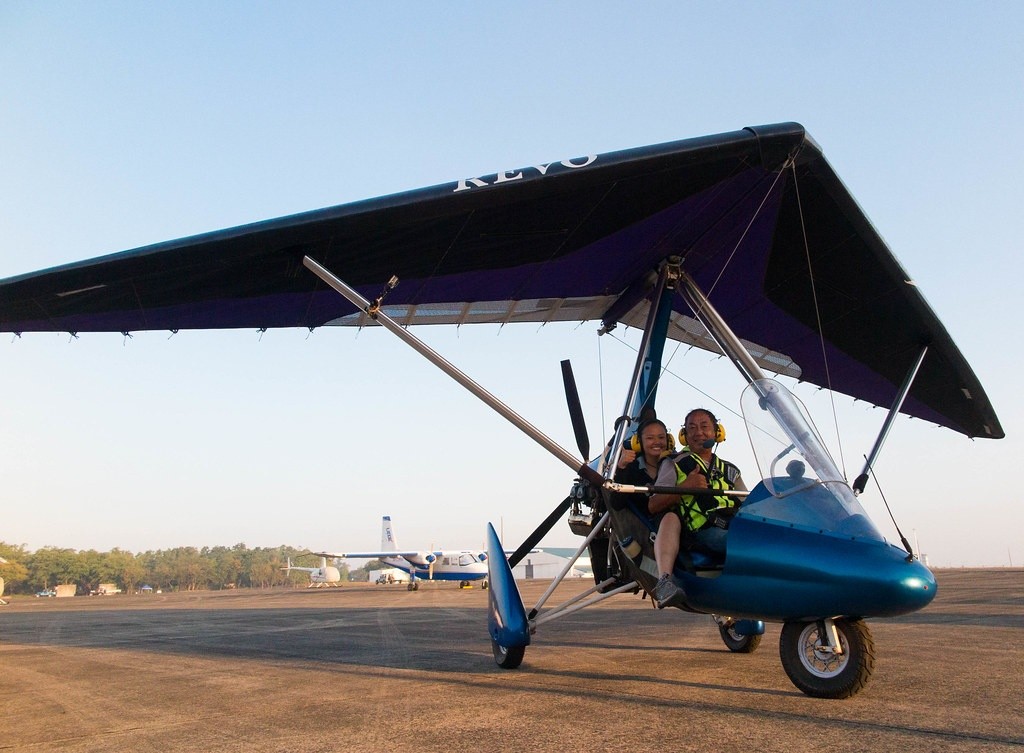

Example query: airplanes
[1,123,1004,701]
[342,516,542,591]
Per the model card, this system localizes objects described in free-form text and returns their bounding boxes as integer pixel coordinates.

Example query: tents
[141,585,152,594]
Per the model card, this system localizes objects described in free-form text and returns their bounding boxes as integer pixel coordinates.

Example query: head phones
[679,408,725,446]
[631,418,675,452]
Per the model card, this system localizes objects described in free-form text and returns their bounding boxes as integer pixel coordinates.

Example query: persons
[611,418,669,511]
[649,409,748,609]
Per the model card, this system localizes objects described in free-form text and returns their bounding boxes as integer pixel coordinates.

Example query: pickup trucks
[37,588,57,597]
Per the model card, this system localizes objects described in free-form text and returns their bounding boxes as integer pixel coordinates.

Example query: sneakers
[655,578,686,609]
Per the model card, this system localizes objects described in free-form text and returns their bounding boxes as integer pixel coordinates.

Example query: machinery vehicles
[376,573,401,584]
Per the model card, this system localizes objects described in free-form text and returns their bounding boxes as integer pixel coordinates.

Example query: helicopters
[278,551,342,589]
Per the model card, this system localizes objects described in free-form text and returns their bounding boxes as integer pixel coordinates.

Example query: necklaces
[644,459,657,469]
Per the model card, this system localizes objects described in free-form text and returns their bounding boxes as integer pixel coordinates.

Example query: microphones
[702,438,716,448]
